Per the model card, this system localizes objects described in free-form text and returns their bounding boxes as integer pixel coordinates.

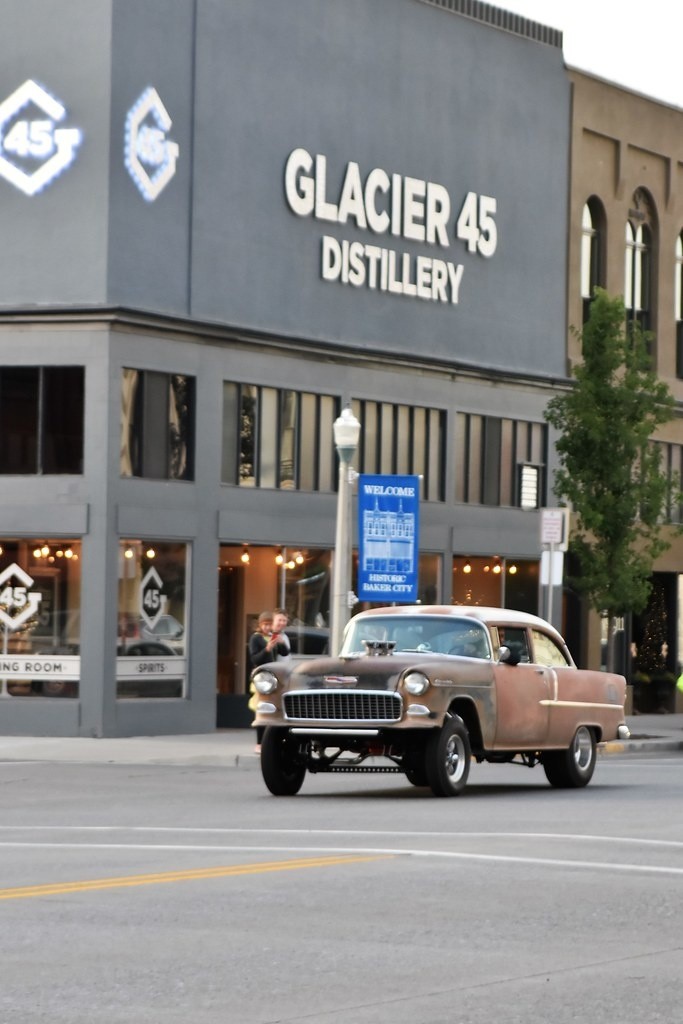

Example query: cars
[14,605,184,655]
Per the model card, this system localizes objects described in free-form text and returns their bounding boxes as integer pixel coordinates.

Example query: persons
[455,626,522,668]
[248,608,291,754]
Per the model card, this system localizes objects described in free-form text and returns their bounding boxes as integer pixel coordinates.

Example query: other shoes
[255,744,262,753]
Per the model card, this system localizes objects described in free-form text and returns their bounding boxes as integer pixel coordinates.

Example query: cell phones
[272,634,278,641]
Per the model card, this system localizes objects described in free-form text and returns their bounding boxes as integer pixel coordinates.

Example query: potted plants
[631,578,677,714]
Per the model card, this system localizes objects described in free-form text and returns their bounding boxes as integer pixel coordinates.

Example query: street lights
[329,406,361,655]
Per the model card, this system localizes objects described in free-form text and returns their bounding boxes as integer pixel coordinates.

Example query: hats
[259,612,273,623]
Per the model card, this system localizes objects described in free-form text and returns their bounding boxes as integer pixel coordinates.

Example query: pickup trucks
[248,605,631,797]
